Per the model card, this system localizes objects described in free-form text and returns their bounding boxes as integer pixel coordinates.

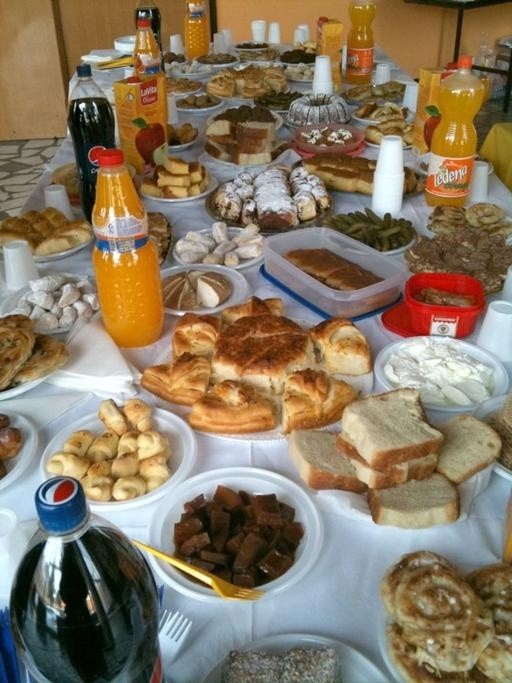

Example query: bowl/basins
[402,271,486,338]
[264,228,404,320]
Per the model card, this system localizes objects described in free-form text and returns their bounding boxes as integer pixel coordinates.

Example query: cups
[44,182,76,222]
[371,136,408,217]
[9,472,166,682]
[2,240,44,292]
[471,160,489,205]
[311,54,333,94]
[475,298,511,365]
[1,506,31,570]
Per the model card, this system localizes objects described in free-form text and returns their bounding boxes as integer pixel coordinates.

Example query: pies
[0,314,71,392]
[380,550,512,683]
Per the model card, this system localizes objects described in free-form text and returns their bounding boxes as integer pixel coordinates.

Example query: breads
[165,64,418,165]
[140,156,211,200]
[211,163,331,231]
[140,297,372,437]
[288,386,502,528]
[161,270,231,311]
[46,398,172,502]
[1,206,92,256]
[301,152,416,194]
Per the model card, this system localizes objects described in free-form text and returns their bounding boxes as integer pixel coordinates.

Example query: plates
[374,334,511,413]
[171,225,266,270]
[200,143,293,172]
[337,94,404,107]
[199,53,241,68]
[168,64,212,81]
[232,41,270,54]
[324,488,373,525]
[205,107,285,132]
[18,233,97,263]
[14,272,104,338]
[376,581,510,683]
[291,130,366,161]
[138,171,219,204]
[285,72,313,85]
[37,405,200,514]
[362,132,414,152]
[417,152,494,180]
[146,464,327,606]
[280,57,315,69]
[205,177,334,234]
[0,352,69,401]
[173,93,226,113]
[1,404,41,492]
[208,75,292,101]
[421,211,512,239]
[323,215,419,258]
[349,106,415,126]
[199,632,392,682]
[160,262,250,320]
[467,394,511,485]
[283,109,349,132]
[148,315,374,446]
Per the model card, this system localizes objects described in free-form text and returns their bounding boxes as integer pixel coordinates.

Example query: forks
[129,538,266,603]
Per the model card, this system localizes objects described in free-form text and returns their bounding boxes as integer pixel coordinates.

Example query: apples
[423,114,443,151]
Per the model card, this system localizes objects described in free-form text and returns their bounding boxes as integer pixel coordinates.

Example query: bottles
[423,53,487,209]
[89,146,171,350]
[183,0,210,59]
[345,0,378,86]
[135,0,166,70]
[66,64,119,224]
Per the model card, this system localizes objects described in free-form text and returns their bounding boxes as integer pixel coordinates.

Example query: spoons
[155,607,196,677]
